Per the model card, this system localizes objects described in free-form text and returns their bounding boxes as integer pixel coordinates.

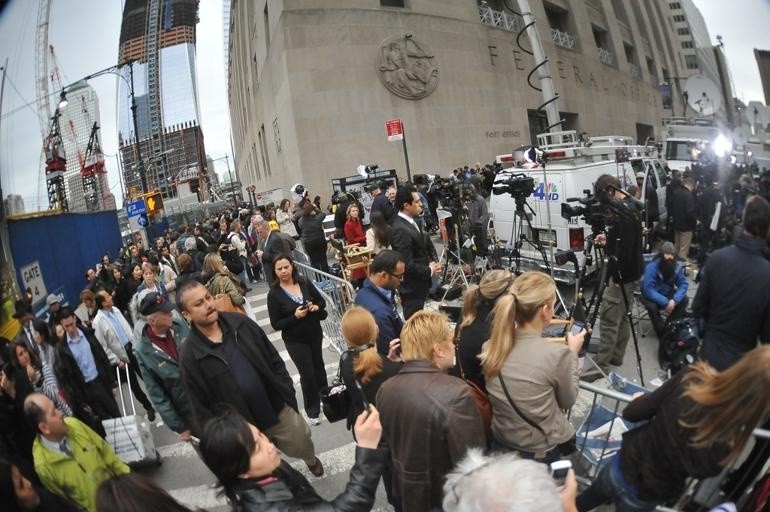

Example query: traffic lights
[145,192,164,213]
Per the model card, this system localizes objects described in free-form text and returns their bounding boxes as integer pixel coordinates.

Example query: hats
[663,242,675,254]
[12,299,33,318]
[139,292,174,316]
[46,293,58,305]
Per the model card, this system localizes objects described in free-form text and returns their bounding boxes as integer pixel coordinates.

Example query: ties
[412,220,420,233]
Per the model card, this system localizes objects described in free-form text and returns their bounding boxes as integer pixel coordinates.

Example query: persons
[0,142,770,511]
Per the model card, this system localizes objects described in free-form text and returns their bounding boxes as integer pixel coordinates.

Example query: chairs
[564,373,652,486]
[335,248,374,309]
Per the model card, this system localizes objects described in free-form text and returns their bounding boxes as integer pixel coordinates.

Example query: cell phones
[354,380,371,411]
[570,321,584,336]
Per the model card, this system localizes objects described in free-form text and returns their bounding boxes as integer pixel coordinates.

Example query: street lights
[56,73,157,239]
[249,184,257,209]
[176,161,201,230]
[245,185,253,208]
[144,146,175,193]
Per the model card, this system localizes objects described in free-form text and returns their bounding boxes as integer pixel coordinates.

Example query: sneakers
[245,287,252,292]
[252,279,258,283]
[309,417,320,425]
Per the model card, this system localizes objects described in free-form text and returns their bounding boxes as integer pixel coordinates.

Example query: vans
[732,151,753,170]
[490,127,678,284]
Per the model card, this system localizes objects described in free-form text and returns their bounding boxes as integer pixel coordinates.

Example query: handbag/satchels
[462,378,492,430]
[309,296,328,320]
[661,322,699,361]
[213,291,247,316]
[319,384,352,423]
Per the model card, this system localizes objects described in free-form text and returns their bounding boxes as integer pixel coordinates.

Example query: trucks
[656,118,734,188]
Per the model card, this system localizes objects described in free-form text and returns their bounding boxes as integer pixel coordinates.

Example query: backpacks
[219,234,239,261]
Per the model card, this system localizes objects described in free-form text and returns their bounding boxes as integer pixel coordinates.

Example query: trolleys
[113,356,162,470]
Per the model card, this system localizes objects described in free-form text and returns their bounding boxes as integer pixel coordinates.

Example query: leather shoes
[308,458,323,476]
[148,408,155,421]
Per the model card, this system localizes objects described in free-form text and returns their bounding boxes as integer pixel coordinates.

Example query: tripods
[509,210,552,277]
[441,218,470,303]
[575,238,649,387]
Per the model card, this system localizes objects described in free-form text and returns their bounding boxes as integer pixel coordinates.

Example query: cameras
[549,459,572,492]
